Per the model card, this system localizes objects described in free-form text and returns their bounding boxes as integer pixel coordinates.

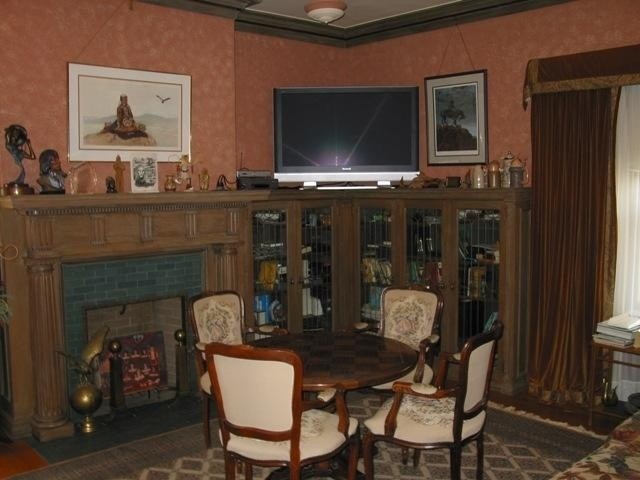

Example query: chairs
[188,288,292,451]
[204,342,362,476]
[364,321,506,475]
[367,282,445,406]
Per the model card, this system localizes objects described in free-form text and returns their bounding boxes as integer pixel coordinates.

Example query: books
[591,313,640,349]
[250,209,500,335]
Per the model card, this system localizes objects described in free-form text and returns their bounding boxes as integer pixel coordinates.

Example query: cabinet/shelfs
[248,188,347,342]
[589,333,640,433]
[344,187,533,397]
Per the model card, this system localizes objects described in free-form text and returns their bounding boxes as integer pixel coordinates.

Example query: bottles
[470,152,531,189]
[600,377,619,408]
[363,237,440,289]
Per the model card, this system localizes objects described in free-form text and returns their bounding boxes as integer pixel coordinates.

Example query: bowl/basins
[627,392,640,418]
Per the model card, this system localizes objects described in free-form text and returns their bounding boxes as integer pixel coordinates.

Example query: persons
[447,100,456,111]
[113,155,126,192]
[114,93,137,133]
[99,345,161,392]
[174,154,203,185]
[35,149,67,191]
[105,176,118,193]
[198,167,210,192]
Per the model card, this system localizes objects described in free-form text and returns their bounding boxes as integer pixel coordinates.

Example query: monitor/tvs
[273,87,419,189]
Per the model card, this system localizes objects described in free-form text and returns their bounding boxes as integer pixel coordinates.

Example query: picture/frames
[128,152,159,194]
[66,61,194,163]
[422,67,491,168]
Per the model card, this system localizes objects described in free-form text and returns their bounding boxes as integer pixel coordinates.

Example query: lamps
[303,1,347,25]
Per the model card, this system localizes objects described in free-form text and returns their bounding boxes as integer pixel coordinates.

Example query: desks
[238,330,421,470]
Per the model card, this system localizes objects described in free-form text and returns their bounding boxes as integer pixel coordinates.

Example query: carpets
[1,390,611,475]
